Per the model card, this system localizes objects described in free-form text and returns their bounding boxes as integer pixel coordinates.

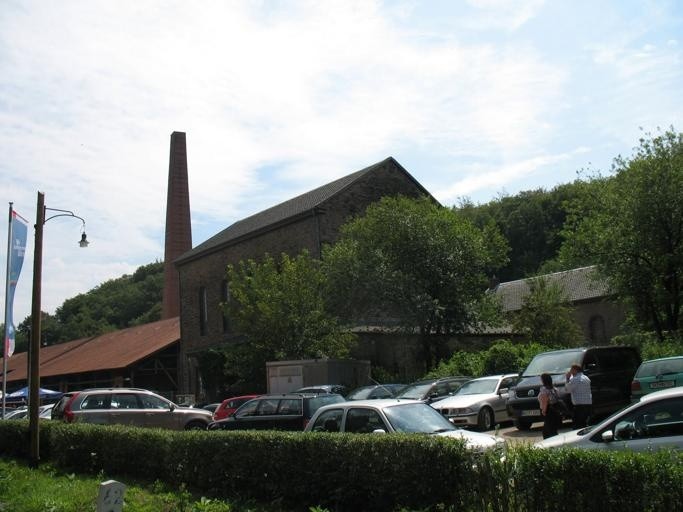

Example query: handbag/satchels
[558,399,568,414]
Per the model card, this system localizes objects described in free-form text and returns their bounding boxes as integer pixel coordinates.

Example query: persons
[564,363,593,430]
[537,373,562,439]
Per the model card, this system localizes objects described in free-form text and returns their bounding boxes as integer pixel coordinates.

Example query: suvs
[51,387,214,433]
[390,375,474,406]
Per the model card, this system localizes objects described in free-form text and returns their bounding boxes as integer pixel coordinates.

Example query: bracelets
[566,378,570,381]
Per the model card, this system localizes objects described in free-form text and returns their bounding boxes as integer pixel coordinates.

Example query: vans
[506,345,644,430]
[204,392,347,432]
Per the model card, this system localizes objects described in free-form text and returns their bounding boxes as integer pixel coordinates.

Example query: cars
[294,384,351,398]
[430,372,519,432]
[345,383,408,400]
[214,394,288,420]
[302,373,515,471]
[630,356,683,402]
[0,403,128,422]
[533,385,683,455]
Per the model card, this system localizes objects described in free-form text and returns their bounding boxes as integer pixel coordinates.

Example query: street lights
[26,190,90,469]
